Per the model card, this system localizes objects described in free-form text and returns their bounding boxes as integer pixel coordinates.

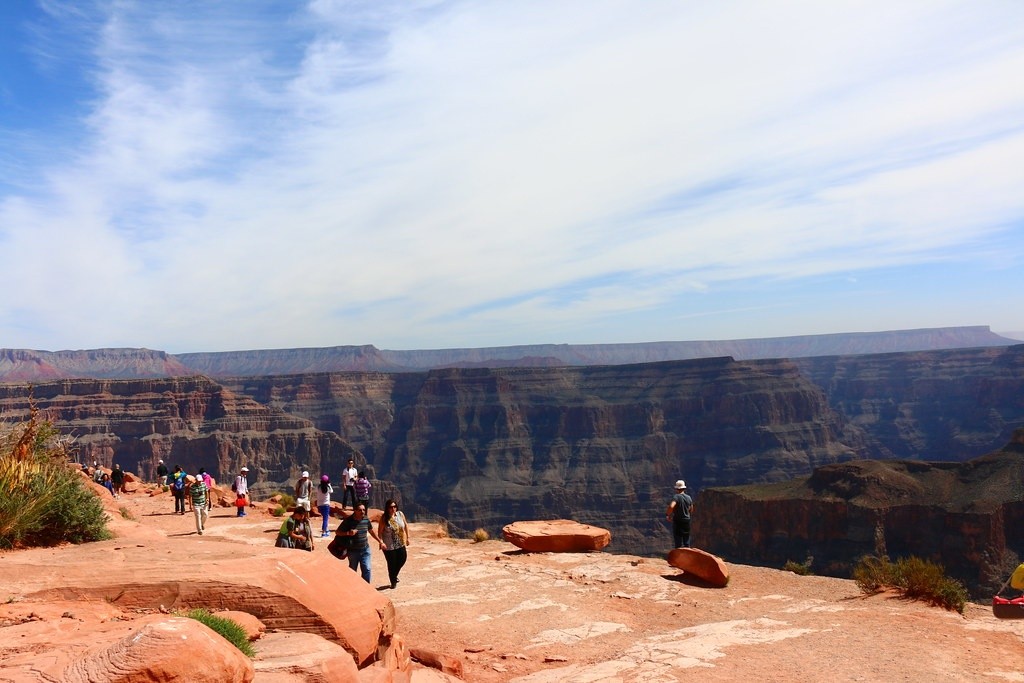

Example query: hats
[302,471,309,478]
[320,475,329,482]
[241,468,249,472]
[196,474,203,481]
[158,460,163,464]
[674,480,687,490]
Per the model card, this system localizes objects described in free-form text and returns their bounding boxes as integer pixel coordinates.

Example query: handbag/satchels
[327,535,351,560]
[276,535,290,549]
[173,480,184,491]
[236,497,249,507]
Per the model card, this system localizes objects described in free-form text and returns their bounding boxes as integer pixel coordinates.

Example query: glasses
[389,505,397,509]
[357,508,365,512]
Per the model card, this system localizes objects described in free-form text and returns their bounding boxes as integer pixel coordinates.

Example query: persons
[378,498,410,589]
[157,459,168,485]
[335,503,388,584]
[277,505,315,551]
[236,467,250,517]
[189,474,209,535]
[353,472,371,516]
[666,480,694,549]
[295,471,313,519]
[341,460,358,510]
[94,464,105,482]
[111,464,124,496]
[168,465,187,515]
[100,473,114,496]
[199,468,212,511]
[81,464,89,476]
[317,475,333,537]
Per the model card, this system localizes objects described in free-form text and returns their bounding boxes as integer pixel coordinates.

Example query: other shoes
[181,511,184,515]
[391,578,401,589]
[202,526,204,530]
[237,512,246,517]
[321,530,330,537]
[199,532,202,535]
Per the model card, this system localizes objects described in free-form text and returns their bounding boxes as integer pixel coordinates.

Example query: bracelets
[666,513,670,516]
[347,530,350,536]
[206,499,209,501]
[407,536,410,538]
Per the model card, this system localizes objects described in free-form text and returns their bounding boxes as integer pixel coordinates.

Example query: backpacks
[232,475,246,492]
